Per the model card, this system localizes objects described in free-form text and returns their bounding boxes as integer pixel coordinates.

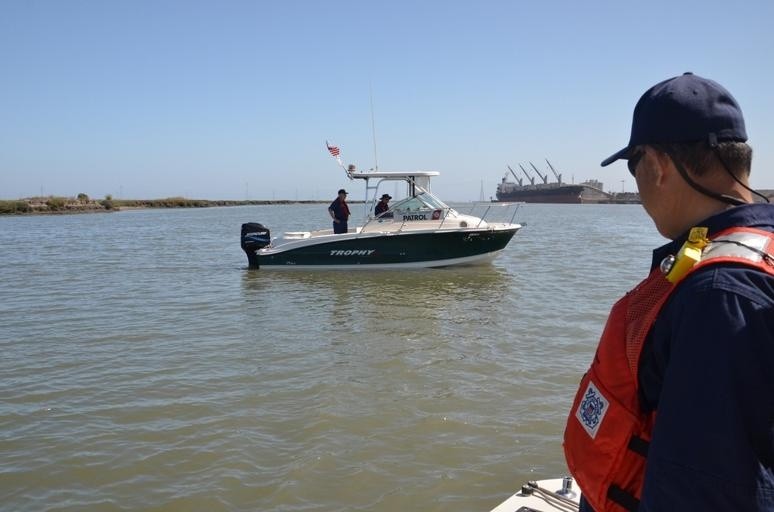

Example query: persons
[374,194,394,220]
[555,72,774,512]
[326,190,351,235]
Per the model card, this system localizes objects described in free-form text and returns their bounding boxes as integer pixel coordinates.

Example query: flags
[325,138,340,159]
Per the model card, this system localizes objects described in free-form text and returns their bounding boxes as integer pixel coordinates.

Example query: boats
[496,159,585,204]
[240,164,526,270]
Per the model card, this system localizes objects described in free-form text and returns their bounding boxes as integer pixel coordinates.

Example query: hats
[379,194,392,199]
[599,71,748,168]
[338,189,349,194]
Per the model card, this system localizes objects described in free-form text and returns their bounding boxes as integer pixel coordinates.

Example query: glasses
[627,147,648,177]
